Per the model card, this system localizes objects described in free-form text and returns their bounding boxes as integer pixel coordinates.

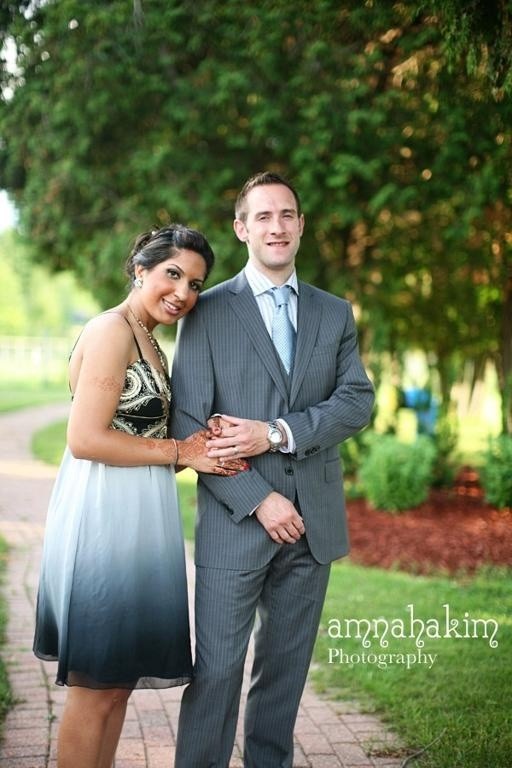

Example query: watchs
[261,420,284,456]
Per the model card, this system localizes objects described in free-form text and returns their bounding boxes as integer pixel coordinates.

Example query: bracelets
[171,438,182,468]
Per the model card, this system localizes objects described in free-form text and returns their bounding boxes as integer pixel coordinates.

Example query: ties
[267,287,297,375]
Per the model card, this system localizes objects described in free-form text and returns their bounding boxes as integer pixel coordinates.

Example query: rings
[220,461,226,468]
[232,445,240,455]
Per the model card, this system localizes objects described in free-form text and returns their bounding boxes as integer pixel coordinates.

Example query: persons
[169,170,376,768]
[31,224,252,768]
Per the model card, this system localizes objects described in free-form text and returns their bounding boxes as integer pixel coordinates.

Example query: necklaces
[123,298,171,383]
